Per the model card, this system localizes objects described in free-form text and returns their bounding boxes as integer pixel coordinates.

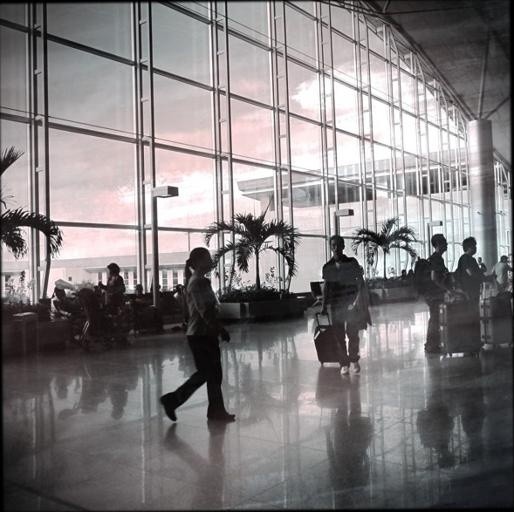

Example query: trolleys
[73,288,123,351]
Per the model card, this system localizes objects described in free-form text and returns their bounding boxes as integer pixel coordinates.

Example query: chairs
[308,282,324,307]
[127,292,182,328]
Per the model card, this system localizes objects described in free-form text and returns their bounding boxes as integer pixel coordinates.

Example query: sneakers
[341,362,360,375]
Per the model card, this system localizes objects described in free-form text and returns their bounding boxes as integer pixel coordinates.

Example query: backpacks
[413,256,432,294]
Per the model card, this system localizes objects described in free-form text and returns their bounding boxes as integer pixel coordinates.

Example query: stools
[10,311,40,357]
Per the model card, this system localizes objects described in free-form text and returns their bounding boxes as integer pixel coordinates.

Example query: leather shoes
[160,393,177,421]
[207,411,236,421]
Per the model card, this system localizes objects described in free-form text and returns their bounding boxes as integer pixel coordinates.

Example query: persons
[420,233,514,350]
[52,261,165,348]
[162,246,236,424]
[317,235,366,374]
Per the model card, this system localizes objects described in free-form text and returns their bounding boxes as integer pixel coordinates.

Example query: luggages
[440,300,481,353]
[485,295,513,345]
[314,312,339,363]
